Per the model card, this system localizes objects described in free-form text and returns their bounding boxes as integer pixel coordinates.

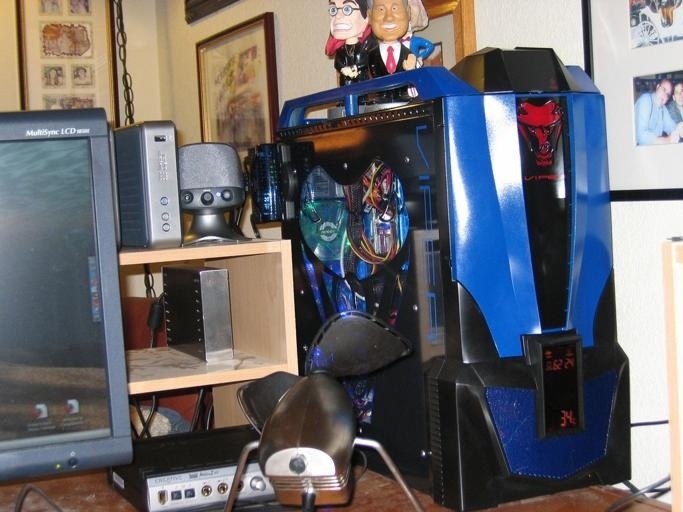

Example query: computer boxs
[277,45,632,511]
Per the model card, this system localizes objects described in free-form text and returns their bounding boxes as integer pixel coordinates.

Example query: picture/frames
[16,0,121,135]
[193,11,279,153]
[581,0,683,201]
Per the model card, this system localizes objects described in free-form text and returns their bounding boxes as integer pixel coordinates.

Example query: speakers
[176,142,254,244]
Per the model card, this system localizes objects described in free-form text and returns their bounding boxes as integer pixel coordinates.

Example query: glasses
[659,85,670,97]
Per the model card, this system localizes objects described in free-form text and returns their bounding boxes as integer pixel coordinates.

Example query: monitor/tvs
[0,107,135,482]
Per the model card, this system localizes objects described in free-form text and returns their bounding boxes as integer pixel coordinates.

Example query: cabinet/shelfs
[114,238,301,431]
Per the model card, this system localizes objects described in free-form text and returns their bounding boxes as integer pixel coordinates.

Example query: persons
[44,68,61,89]
[325,0,434,119]
[74,67,92,85]
[634,79,683,146]
[661,81,683,143]
